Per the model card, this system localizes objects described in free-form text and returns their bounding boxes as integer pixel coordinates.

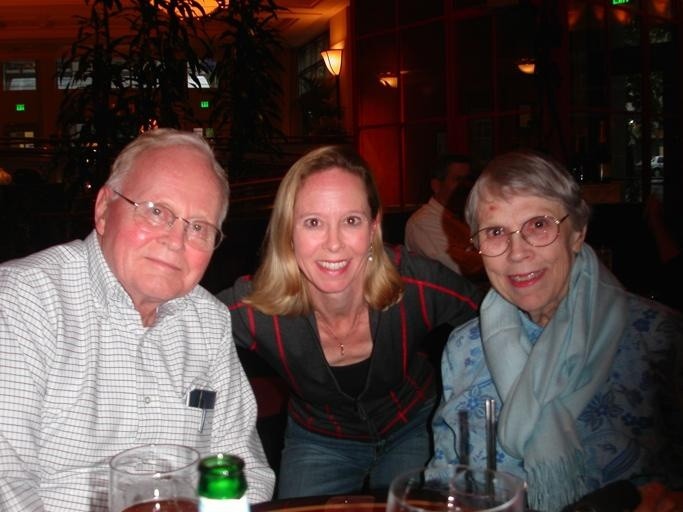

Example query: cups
[104,442,206,512]
[382,462,527,512]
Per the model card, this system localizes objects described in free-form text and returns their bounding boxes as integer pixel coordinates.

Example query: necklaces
[315,311,362,356]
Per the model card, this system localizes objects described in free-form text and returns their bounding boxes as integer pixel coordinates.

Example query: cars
[635,154,664,179]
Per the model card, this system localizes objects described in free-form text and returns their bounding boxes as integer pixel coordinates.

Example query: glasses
[107,188,230,253]
[468,211,571,259]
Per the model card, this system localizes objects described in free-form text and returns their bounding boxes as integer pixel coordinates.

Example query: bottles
[195,453,253,512]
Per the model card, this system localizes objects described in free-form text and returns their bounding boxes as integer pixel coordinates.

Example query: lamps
[318,48,343,135]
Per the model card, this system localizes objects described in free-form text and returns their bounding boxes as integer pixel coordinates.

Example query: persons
[209,144,483,500]
[0,128,276,512]
[422,152,683,512]
[405,157,484,279]
[645,192,683,311]
